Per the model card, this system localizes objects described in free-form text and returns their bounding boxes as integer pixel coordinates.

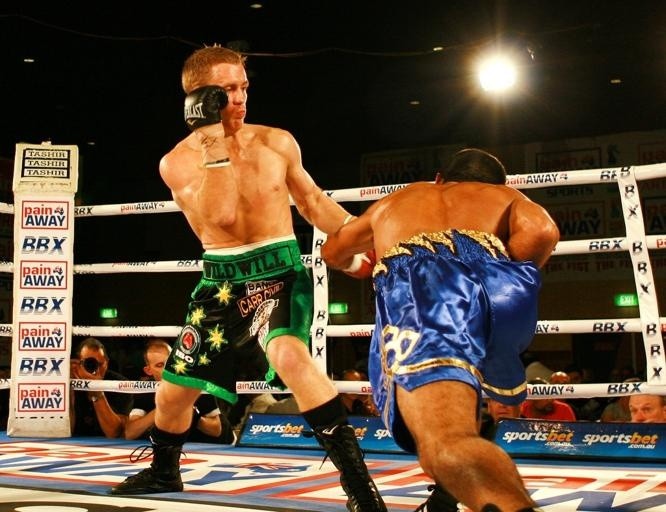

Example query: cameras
[80,356,99,374]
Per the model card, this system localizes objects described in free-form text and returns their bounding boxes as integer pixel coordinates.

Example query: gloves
[182,85,228,128]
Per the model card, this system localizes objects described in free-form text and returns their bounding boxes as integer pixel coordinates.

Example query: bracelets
[343,214,354,225]
[203,157,232,169]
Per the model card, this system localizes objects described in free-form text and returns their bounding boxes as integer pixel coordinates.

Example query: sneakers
[414,491,459,512]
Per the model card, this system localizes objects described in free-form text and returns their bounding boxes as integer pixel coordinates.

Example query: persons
[318,145,563,512]
[106,40,399,510]
[70,334,666,441]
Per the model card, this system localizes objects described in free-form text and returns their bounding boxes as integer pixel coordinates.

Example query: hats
[525,361,552,385]
[551,372,570,384]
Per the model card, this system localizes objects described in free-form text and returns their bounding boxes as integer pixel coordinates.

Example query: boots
[311,426,390,512]
[106,428,190,495]
